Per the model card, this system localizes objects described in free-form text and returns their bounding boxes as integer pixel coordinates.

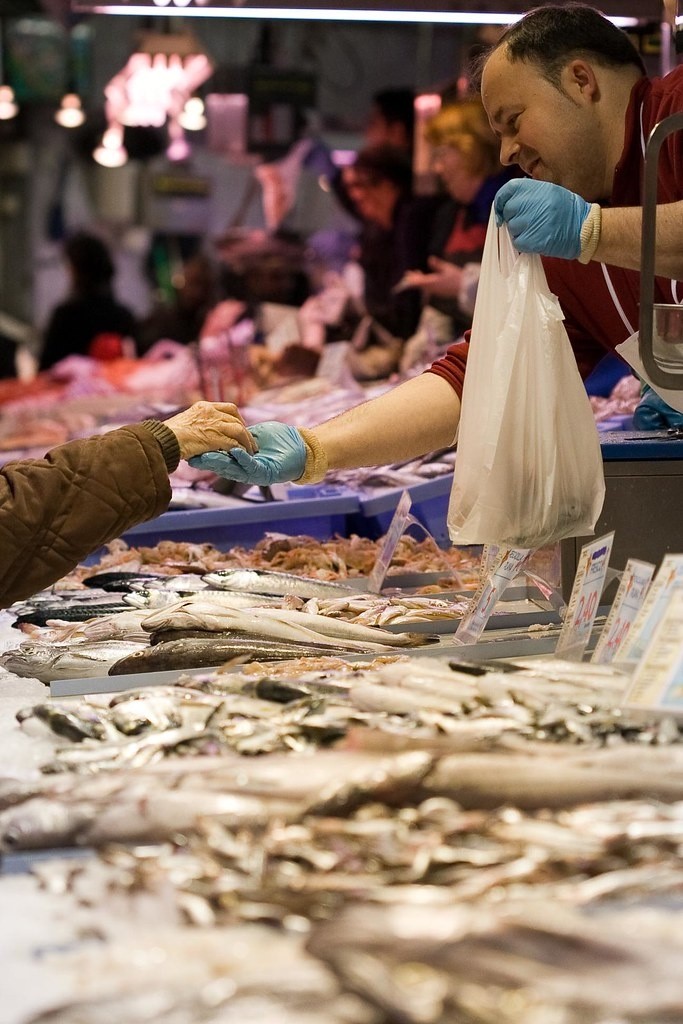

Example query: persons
[311,86,526,368]
[187,6,683,487]
[1,401,258,609]
[40,230,308,373]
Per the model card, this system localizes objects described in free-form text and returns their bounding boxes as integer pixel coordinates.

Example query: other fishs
[0,569,682,1024]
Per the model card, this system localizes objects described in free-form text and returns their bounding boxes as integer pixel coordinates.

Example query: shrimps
[56,530,491,593]
